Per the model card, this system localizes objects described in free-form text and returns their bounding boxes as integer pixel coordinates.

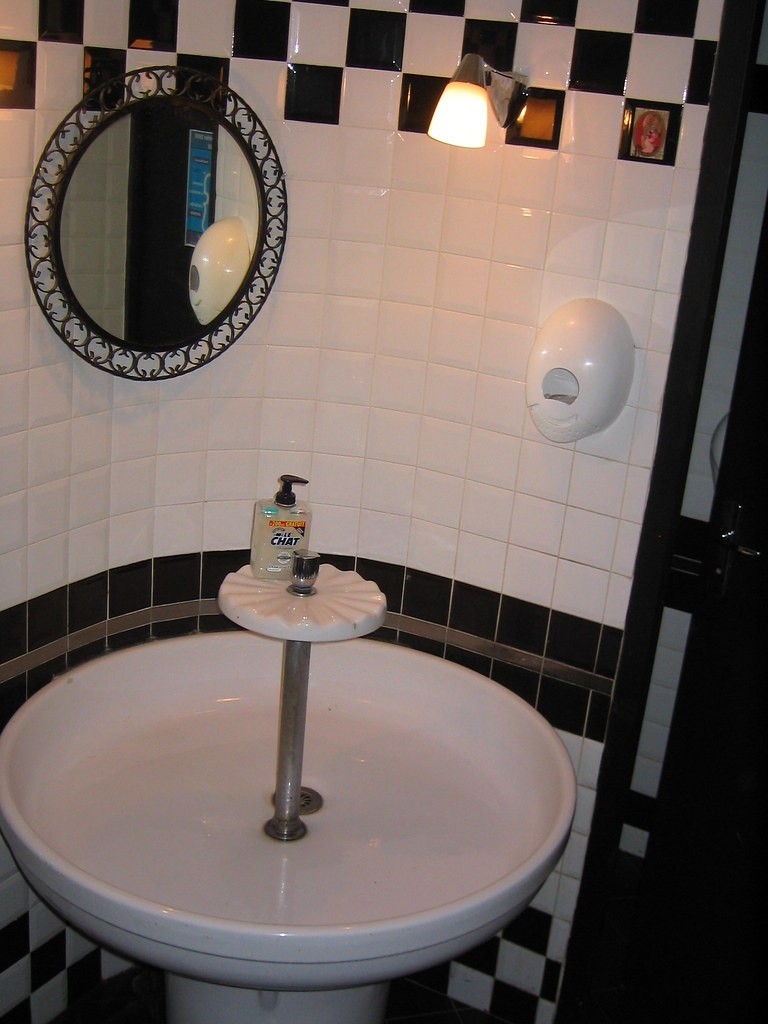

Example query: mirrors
[25,66,288,381]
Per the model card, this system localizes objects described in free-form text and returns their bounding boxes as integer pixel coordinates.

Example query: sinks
[0,630,586,995]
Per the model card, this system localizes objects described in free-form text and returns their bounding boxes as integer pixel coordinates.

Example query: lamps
[426,52,529,148]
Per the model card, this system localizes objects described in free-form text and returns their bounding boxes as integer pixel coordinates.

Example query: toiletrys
[250,471,312,578]
